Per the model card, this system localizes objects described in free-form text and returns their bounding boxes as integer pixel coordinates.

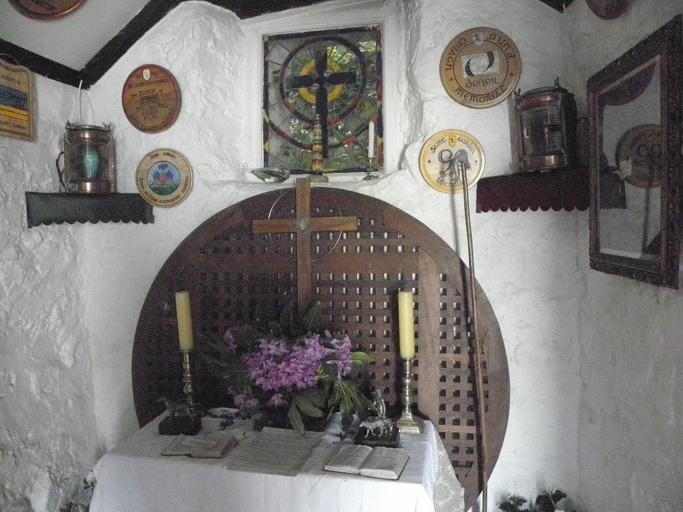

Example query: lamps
[514,76,579,173]
[61,123,114,192]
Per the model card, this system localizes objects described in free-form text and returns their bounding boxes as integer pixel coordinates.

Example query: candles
[398,291,414,360]
[173,290,194,352]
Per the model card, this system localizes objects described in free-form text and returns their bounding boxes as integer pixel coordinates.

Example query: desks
[87,403,465,512]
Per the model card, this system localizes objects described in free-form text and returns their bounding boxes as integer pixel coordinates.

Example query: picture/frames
[585,15,683,286]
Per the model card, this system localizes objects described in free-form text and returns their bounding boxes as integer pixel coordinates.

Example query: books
[160,431,237,457]
[322,443,410,481]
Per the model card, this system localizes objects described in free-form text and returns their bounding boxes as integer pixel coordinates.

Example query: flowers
[218,322,355,413]
